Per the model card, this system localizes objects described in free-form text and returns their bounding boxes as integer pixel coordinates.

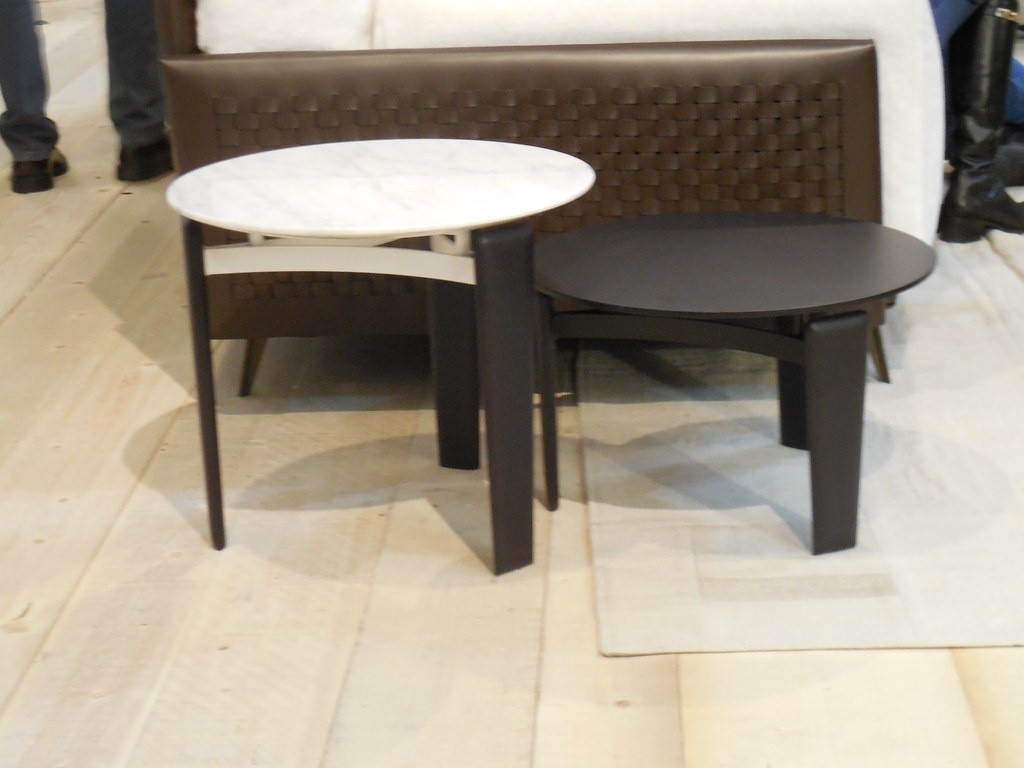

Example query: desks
[532,210,938,557]
[165,139,596,576]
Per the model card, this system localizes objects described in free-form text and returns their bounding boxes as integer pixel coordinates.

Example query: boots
[930,0,1024,242]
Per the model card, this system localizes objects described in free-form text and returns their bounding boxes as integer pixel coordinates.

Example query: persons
[0,0,174,194]
[928,0,1024,243]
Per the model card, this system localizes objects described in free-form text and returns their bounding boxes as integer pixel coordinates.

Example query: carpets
[573,239,1024,657]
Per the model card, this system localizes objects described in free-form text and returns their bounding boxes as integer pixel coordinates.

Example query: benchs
[160,41,899,400]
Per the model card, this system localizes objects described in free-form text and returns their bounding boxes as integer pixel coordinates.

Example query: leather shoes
[118,135,173,181]
[13,148,67,194]
